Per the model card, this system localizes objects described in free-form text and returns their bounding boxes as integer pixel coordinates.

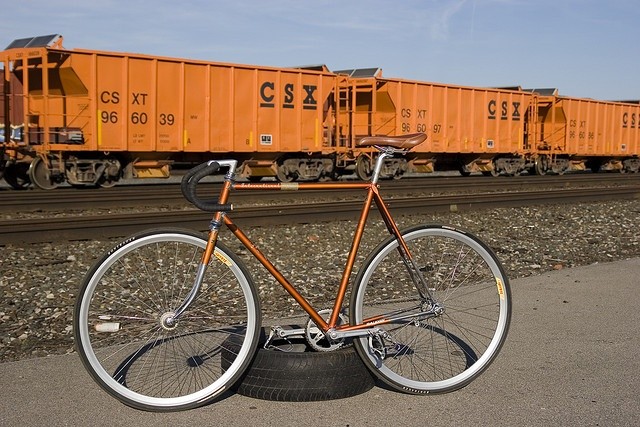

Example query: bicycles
[74,133,511,413]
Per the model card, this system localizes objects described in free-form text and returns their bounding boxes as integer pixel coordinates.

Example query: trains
[0,33,640,191]
